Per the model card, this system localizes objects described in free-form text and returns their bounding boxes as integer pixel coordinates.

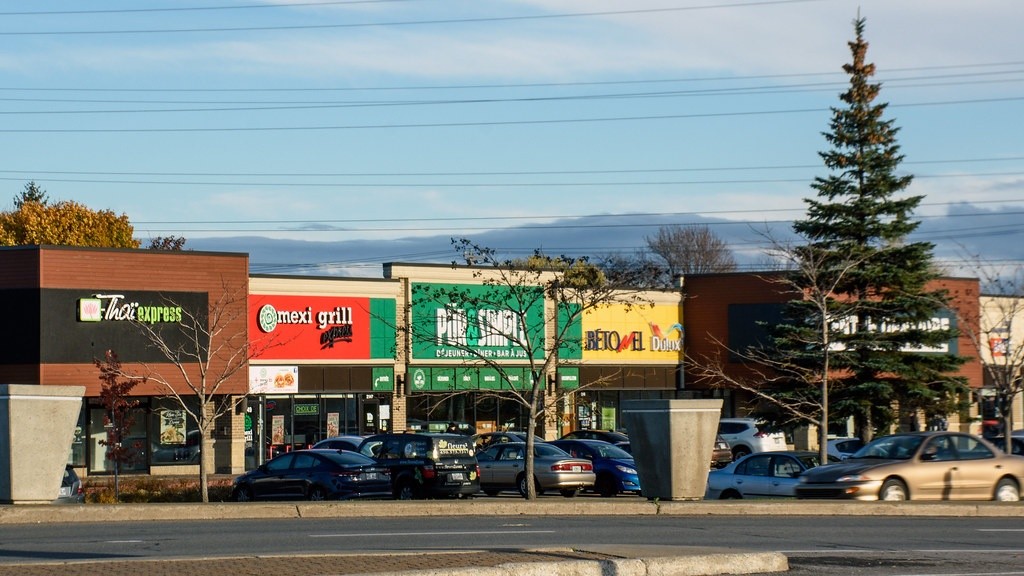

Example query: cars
[827,438,908,460]
[311,436,364,452]
[58,464,85,504]
[474,442,597,498]
[232,449,392,500]
[560,430,630,454]
[705,451,841,500]
[711,434,733,470]
[470,432,545,453]
[546,440,642,497]
[794,430,1024,501]
[718,417,787,462]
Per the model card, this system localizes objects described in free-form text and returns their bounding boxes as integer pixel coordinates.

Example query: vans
[356,433,480,500]
